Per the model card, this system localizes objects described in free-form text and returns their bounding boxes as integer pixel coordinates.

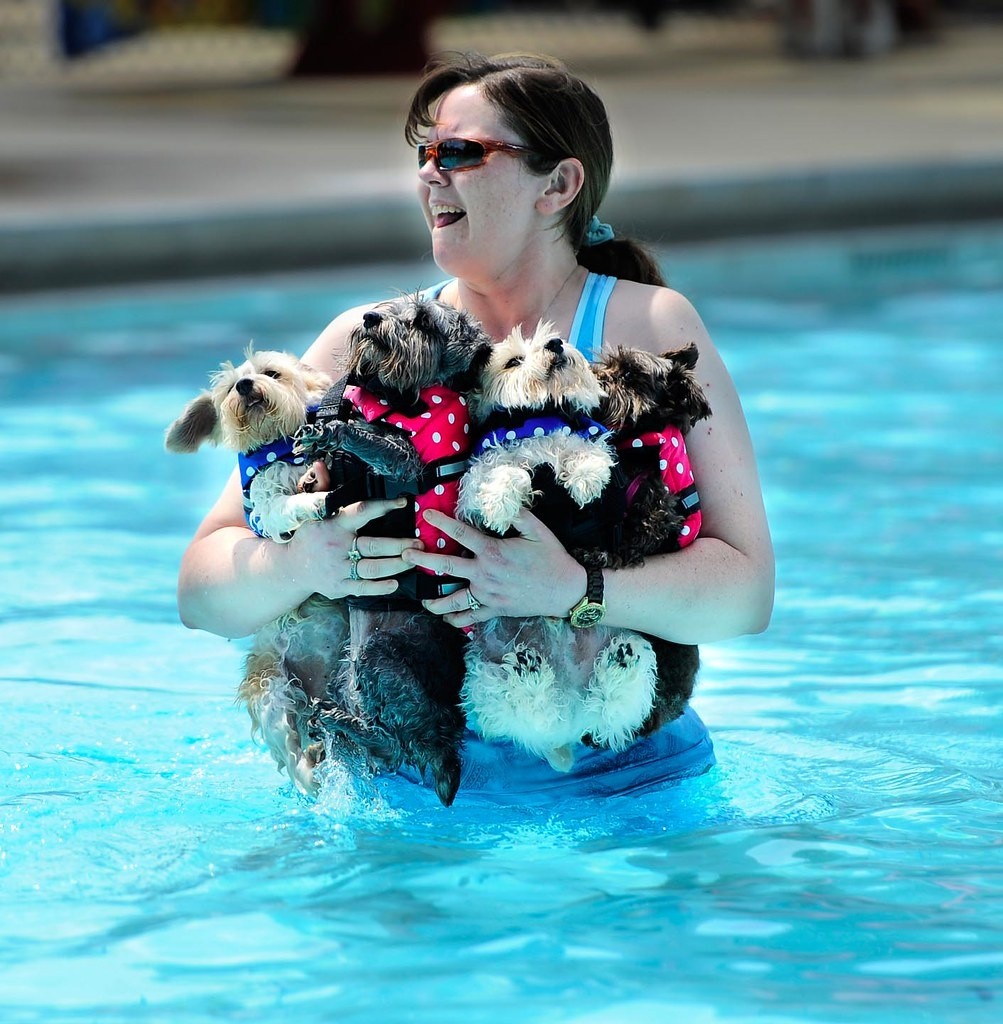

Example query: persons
[176,51,774,821]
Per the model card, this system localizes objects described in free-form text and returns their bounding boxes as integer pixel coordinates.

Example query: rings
[347,536,362,561]
[465,587,481,611]
[350,560,362,580]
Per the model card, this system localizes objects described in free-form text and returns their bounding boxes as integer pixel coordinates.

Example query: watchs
[565,564,606,631]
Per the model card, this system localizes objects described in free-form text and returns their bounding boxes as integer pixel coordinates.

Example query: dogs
[453,318,659,777]
[163,337,350,797]
[294,283,493,807]
[586,340,714,737]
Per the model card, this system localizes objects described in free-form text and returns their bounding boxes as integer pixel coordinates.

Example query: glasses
[417,136,538,172]
